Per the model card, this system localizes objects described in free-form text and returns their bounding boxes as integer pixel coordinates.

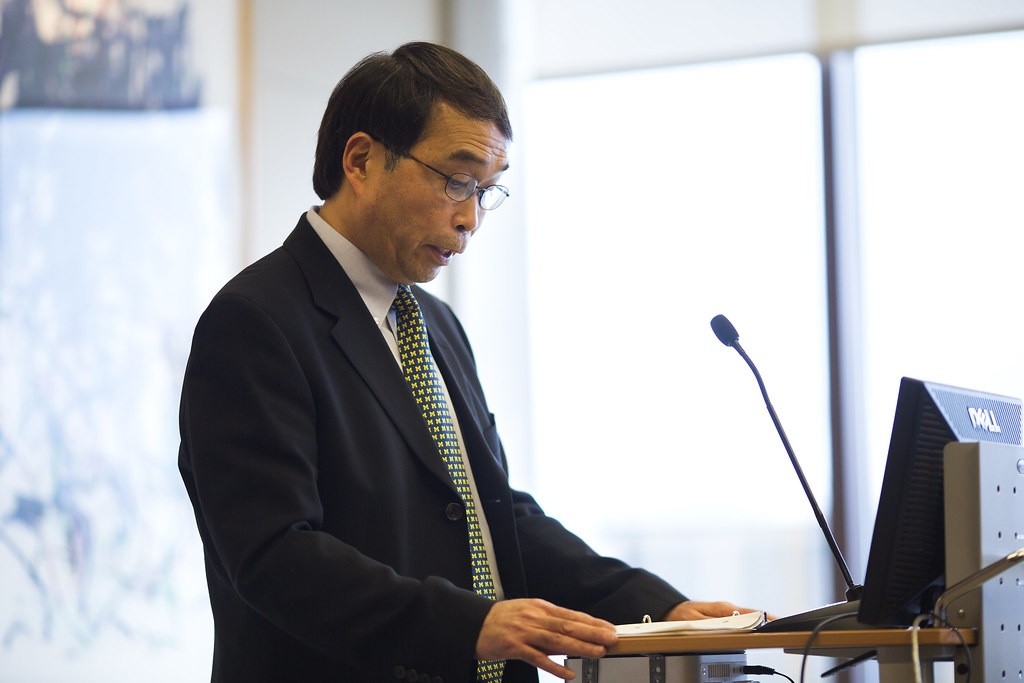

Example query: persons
[179,40,775,683]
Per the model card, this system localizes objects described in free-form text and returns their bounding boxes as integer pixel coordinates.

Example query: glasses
[369,136,510,210]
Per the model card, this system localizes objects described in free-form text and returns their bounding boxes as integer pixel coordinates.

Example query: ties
[390,283,506,683]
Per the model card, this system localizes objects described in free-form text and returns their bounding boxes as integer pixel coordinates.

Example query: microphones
[710,314,878,631]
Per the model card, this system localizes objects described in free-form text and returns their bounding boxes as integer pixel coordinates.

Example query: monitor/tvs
[856,377,1024,626]
